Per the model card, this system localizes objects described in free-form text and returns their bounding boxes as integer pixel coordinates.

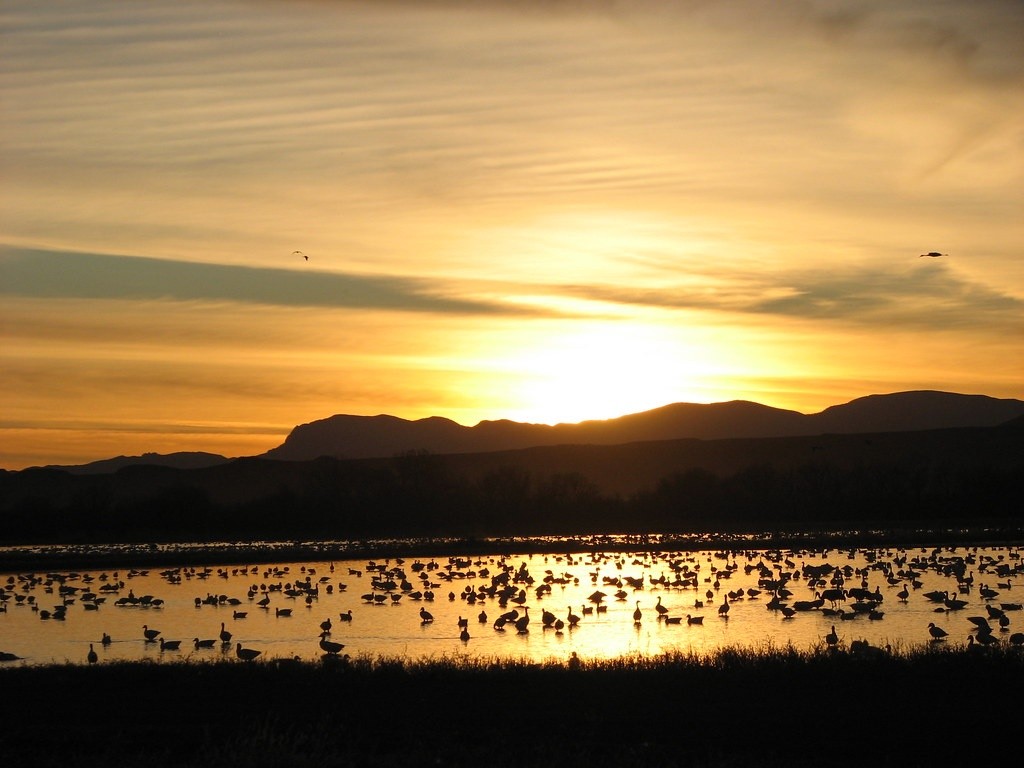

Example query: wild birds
[0,534,1024,672]
[917,252,950,257]
[291,248,310,262]
